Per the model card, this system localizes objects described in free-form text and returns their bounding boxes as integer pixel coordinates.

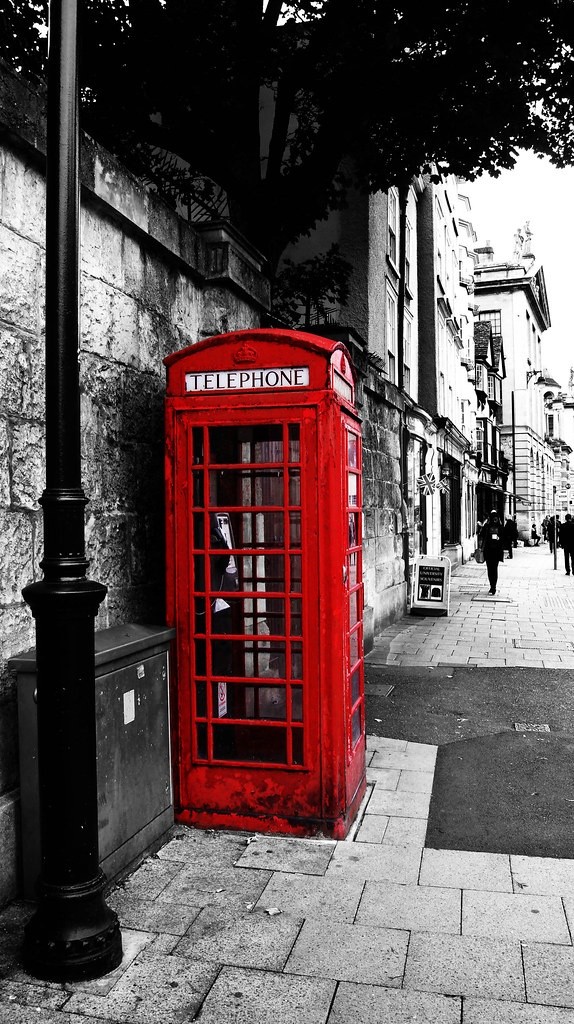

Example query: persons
[531,524,541,546]
[478,510,507,596]
[542,514,574,576]
[503,514,517,559]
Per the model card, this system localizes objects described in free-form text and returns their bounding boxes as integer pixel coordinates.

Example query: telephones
[196,512,240,592]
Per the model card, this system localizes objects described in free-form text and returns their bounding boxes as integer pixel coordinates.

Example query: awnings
[479,481,527,501]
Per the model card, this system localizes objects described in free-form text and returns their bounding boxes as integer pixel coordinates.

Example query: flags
[417,473,436,495]
[436,479,451,494]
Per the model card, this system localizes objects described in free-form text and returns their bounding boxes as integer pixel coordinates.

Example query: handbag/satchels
[475,549,485,563]
